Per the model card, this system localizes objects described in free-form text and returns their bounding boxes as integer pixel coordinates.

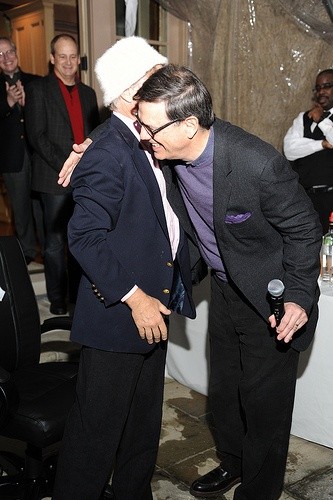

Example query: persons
[50,35,197,500]
[57,65,321,500]
[25,34,101,316]
[0,36,42,266]
[284,68,333,238]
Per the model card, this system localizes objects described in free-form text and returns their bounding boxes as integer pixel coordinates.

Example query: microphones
[267,278,286,345]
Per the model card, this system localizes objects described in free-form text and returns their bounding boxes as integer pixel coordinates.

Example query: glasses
[0,49,16,56]
[131,103,186,139]
[312,84,333,93]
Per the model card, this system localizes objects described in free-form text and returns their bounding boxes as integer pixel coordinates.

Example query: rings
[295,324,298,327]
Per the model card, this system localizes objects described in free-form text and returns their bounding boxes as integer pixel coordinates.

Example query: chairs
[0,236,115,500]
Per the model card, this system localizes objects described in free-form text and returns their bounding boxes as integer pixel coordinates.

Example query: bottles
[321,212,333,284]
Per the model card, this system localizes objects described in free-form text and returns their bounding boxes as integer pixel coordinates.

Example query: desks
[165,271,333,449]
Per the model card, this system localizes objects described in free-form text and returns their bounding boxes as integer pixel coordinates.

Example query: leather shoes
[189,465,241,498]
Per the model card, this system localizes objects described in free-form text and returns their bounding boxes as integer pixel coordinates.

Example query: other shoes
[50,301,67,315]
[25,255,34,265]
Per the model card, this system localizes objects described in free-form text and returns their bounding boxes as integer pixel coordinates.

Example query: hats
[94,36,168,107]
